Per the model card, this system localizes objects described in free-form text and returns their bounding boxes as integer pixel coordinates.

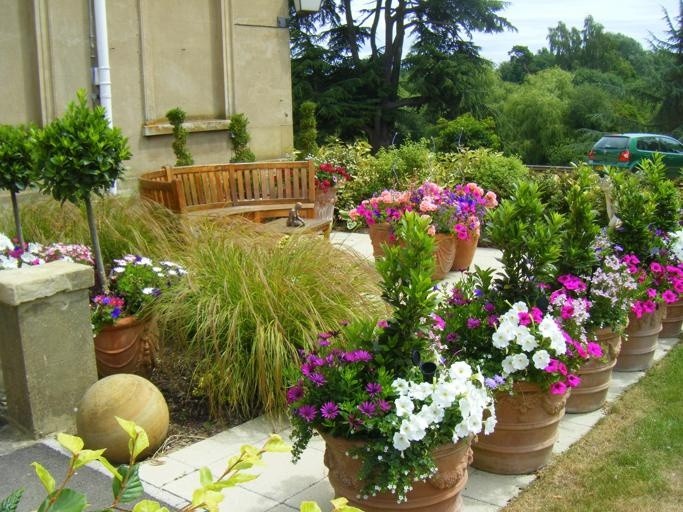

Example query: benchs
[136,158,316,234]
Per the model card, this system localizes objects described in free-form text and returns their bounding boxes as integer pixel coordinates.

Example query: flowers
[91,251,189,341]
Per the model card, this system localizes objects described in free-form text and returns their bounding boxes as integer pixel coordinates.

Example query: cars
[584,132,682,176]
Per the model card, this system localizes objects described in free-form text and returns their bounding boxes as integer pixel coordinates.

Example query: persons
[285,201,307,228]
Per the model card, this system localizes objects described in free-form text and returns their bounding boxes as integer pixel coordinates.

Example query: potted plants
[32,82,190,380]
[285,147,683,511]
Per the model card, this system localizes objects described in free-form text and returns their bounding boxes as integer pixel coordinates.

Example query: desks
[263,216,334,243]
